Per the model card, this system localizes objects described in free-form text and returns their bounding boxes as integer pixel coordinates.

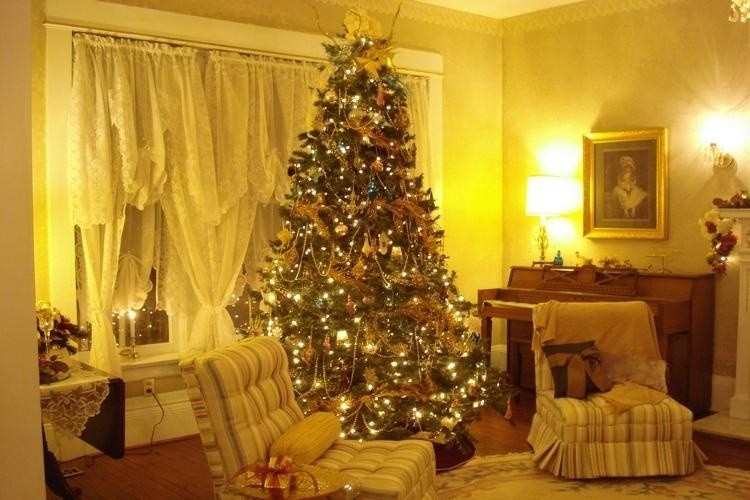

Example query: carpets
[436,451,750,500]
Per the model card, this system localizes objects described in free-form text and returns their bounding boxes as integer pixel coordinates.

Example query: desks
[39,356,125,500]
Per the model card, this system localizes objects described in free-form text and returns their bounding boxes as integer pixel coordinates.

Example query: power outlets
[142,378,155,396]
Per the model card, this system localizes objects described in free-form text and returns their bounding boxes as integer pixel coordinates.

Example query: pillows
[269,411,342,467]
[542,340,612,399]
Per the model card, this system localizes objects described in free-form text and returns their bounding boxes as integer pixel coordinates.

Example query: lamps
[700,113,741,167]
[527,176,578,267]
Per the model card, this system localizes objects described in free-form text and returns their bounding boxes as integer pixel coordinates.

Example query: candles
[128,312,135,338]
[120,311,125,330]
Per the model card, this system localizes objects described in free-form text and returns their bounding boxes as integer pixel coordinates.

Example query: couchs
[526,301,708,480]
[179,336,436,500]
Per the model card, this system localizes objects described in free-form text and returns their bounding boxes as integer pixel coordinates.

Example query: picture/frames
[583,127,670,240]
